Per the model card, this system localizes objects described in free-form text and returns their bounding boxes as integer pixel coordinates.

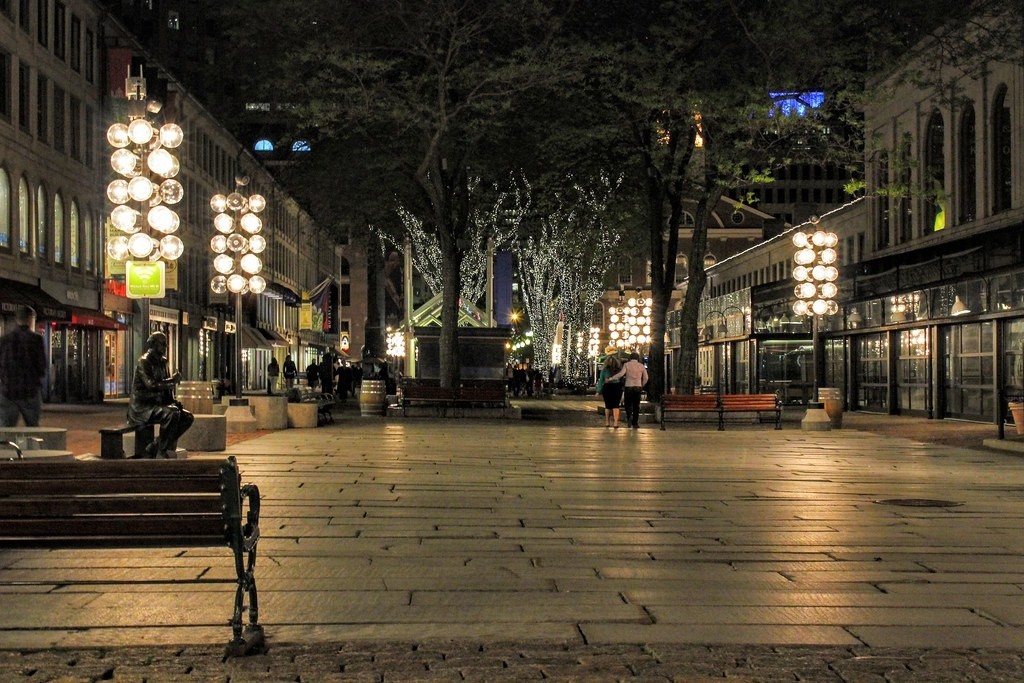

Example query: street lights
[101,51,185,357]
[208,169,268,406]
[576,326,601,390]
[607,286,654,366]
[385,326,406,386]
[793,212,839,409]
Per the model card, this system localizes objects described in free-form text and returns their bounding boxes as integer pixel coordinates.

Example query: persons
[506,363,542,399]
[0,305,46,427]
[127,330,194,459]
[596,353,649,429]
[267,354,363,402]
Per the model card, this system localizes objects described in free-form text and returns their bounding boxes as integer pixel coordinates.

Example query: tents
[596,351,646,363]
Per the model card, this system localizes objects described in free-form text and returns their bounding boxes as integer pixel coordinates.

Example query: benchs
[0,455,262,657]
[660,394,783,430]
[293,388,336,427]
[98,421,155,457]
[400,379,507,419]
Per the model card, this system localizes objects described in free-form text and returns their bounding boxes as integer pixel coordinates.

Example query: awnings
[241,326,273,350]
[258,329,289,348]
[38,305,128,333]
[0,278,72,322]
[309,342,348,358]
[262,280,302,308]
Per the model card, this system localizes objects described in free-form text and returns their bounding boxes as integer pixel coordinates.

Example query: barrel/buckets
[359,380,386,417]
[176,381,213,414]
[818,388,843,428]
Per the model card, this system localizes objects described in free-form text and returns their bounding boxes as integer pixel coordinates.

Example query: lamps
[718,307,744,333]
[700,311,723,336]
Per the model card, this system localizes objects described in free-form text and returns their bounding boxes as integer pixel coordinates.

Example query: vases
[1008,401,1024,435]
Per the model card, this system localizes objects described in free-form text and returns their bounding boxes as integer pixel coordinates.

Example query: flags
[310,286,332,331]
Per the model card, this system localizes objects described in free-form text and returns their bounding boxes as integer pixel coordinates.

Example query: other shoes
[605,424,610,427]
[633,425,639,428]
[614,426,619,428]
[627,415,633,428]
